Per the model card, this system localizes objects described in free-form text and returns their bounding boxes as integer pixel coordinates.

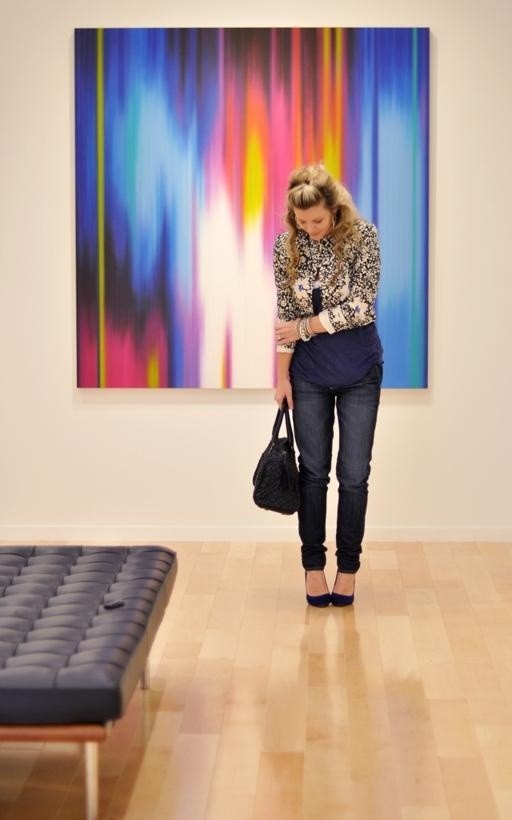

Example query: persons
[273,164,385,608]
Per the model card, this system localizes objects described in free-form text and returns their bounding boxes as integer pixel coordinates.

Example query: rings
[277,335,280,340]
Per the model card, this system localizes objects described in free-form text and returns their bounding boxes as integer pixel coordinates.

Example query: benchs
[0,542,179,820]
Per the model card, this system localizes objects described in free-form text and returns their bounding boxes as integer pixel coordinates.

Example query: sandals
[331,566,357,607]
[304,562,331,608]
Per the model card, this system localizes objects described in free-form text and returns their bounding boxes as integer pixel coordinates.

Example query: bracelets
[296,314,319,343]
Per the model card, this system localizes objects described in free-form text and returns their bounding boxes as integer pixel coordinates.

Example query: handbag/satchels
[250,395,306,516]
